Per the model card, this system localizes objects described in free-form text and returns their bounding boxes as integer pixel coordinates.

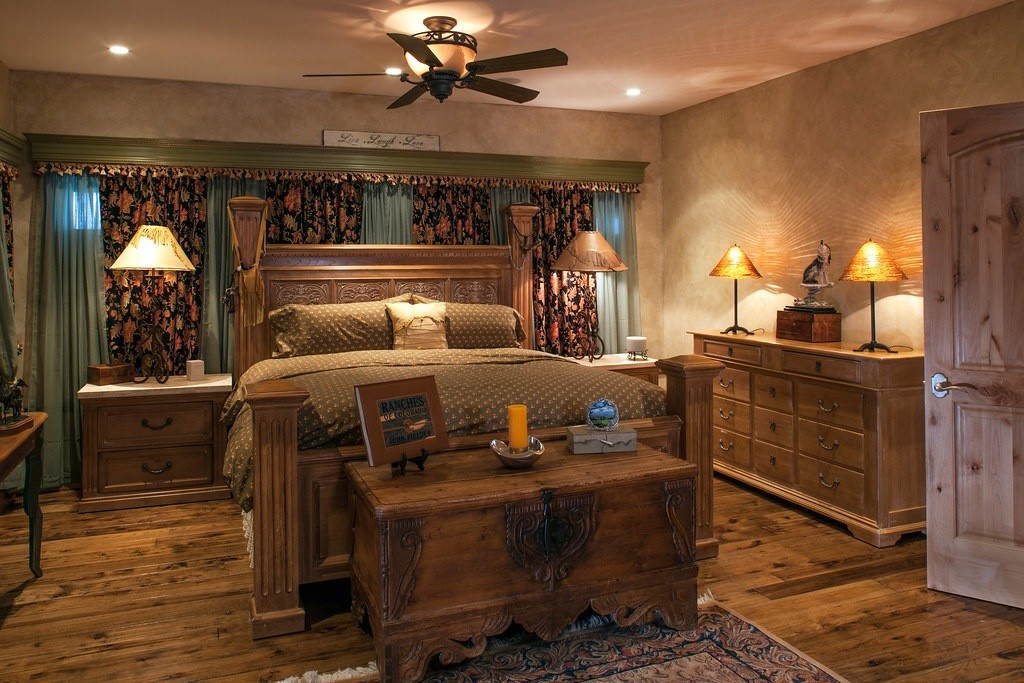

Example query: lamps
[403,17,477,76]
[109,226,196,386]
[710,245,762,336]
[839,239,908,353]
[550,229,629,365]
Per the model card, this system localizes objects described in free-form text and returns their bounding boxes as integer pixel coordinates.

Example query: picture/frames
[355,376,448,467]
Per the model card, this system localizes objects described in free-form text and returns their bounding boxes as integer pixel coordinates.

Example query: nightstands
[75,374,235,511]
[573,353,660,386]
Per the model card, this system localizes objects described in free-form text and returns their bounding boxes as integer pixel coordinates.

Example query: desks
[0,411,49,578]
[345,440,698,683]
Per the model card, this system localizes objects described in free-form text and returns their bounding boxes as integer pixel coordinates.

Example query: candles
[624,336,650,352]
[508,404,528,449]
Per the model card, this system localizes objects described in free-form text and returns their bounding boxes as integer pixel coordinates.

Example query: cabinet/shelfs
[688,331,924,548]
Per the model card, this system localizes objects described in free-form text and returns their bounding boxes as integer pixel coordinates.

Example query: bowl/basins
[491,436,545,468]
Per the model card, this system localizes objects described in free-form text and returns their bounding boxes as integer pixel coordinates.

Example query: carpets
[275,597,848,683]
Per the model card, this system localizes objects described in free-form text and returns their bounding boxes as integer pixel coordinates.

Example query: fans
[301,33,568,110]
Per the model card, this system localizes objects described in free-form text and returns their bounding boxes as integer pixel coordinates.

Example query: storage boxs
[87,363,135,386]
[776,311,842,343]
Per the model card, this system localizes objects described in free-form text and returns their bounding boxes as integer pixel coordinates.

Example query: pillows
[413,295,525,349]
[267,292,412,356]
[382,302,448,351]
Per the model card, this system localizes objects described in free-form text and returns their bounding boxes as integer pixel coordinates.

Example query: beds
[226,195,725,641]
